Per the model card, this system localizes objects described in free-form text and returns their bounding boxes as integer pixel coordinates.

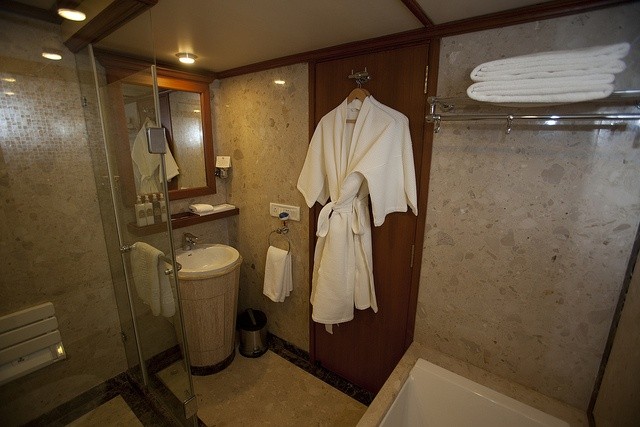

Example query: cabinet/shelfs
[426,89,639,139]
[127,203,239,237]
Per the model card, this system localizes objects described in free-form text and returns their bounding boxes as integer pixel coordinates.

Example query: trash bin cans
[238,307,269,358]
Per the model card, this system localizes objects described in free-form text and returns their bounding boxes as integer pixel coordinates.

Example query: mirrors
[105,57,216,210]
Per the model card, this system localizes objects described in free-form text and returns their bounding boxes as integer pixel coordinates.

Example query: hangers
[345,88,371,123]
[142,115,155,130]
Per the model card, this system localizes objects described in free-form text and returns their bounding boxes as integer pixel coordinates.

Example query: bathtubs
[378,356,573,427]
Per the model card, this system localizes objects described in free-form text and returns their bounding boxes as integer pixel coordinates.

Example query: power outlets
[269,202,301,222]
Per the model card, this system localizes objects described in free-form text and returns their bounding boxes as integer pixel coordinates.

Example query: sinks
[178,247,234,271]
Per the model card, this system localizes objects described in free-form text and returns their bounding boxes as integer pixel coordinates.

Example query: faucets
[182,232,198,250]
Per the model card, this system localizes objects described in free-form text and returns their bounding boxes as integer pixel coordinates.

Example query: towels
[262,246,293,302]
[465,76,615,108]
[467,42,631,81]
[129,242,175,318]
